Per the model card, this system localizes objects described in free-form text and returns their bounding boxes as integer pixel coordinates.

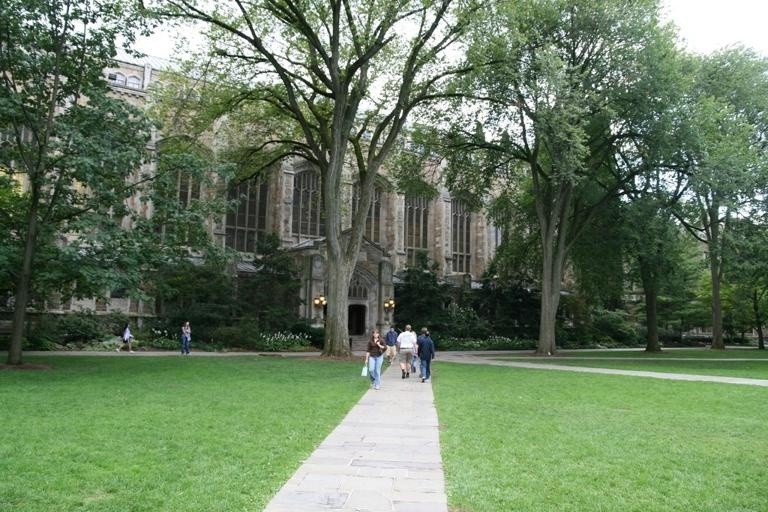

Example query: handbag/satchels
[361,363,368,376]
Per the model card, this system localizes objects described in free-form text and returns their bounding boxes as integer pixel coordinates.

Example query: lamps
[384,296,394,313]
[315,296,326,309]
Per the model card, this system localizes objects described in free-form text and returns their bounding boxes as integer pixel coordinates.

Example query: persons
[114,322,136,354]
[396,324,435,382]
[385,325,398,365]
[364,329,386,390]
[179,320,191,355]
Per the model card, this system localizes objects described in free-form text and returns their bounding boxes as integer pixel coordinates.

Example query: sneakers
[116,349,134,354]
[422,378,425,383]
[372,384,379,390]
[402,364,415,378]
